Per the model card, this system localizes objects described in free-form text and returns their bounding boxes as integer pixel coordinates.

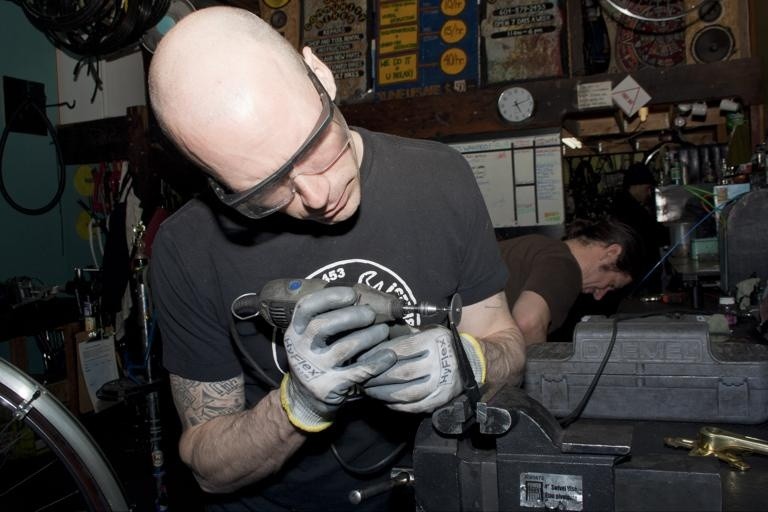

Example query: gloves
[356,316,487,414]
[280,287,397,432]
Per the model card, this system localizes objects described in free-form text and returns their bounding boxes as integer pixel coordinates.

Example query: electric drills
[232,279,452,401]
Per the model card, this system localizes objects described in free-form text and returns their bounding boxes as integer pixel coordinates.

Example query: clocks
[498,87,535,123]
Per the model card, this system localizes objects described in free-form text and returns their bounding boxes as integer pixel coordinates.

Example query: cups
[691,101,707,116]
[719,97,740,114]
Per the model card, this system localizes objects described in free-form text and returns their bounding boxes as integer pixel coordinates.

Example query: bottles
[670,153,682,185]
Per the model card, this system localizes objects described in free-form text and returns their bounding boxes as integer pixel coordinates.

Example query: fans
[140,1,197,55]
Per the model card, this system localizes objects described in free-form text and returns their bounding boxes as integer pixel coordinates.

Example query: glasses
[207,58,350,220]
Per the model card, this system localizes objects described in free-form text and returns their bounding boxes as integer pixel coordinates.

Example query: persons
[148,3,525,512]
[489,209,642,349]
[613,162,675,317]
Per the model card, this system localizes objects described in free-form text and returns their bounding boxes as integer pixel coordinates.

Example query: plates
[678,104,691,112]
[674,117,686,127]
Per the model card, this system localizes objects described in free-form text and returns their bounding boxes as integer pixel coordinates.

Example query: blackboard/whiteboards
[418,126,566,228]
